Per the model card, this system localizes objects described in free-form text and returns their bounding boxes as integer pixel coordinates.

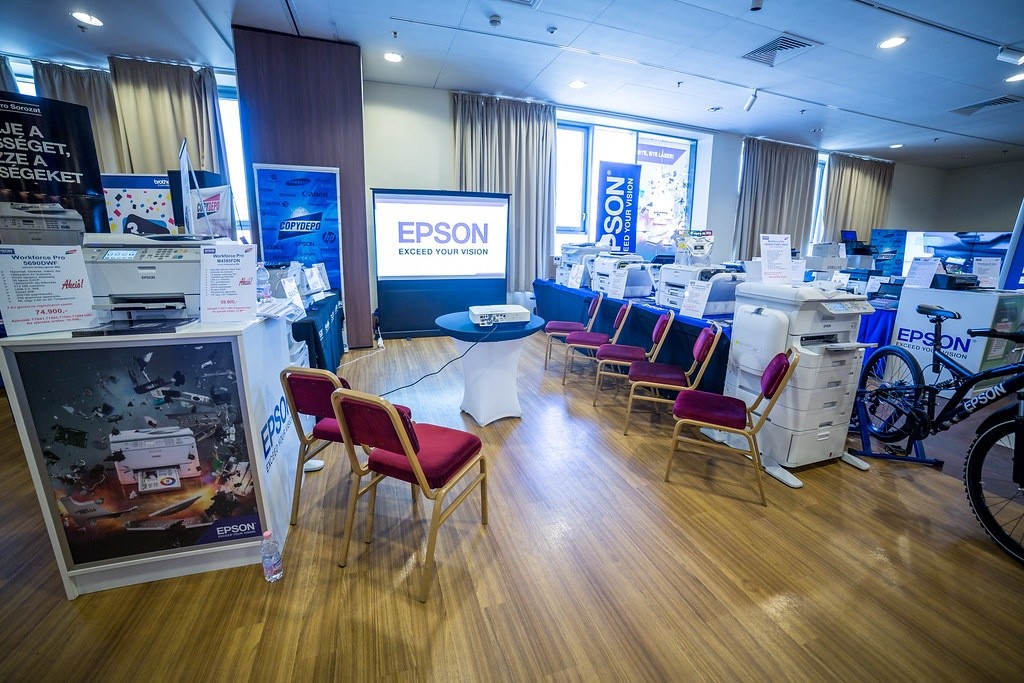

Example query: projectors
[469,305,530,324]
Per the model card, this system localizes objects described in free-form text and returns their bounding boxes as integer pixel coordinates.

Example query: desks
[292,288,346,376]
[435,312,545,427]
[858,298,899,376]
[532,277,733,400]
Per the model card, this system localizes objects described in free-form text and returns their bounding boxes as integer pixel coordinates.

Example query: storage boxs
[846,254,873,269]
[810,240,839,257]
[806,257,849,270]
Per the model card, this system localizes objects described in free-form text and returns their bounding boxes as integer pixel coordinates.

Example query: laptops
[840,230,867,242]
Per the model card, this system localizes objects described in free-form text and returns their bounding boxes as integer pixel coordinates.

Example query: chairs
[330,388,489,604]
[279,368,421,543]
[592,310,675,414]
[624,322,722,447]
[664,345,801,506]
[544,291,603,371]
[562,299,633,392]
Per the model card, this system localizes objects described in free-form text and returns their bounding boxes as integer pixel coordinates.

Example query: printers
[0,201,86,245]
[109,426,202,485]
[560,241,995,488]
[81,233,324,471]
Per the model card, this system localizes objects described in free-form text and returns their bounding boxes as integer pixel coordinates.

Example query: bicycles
[853,307,1024,567]
